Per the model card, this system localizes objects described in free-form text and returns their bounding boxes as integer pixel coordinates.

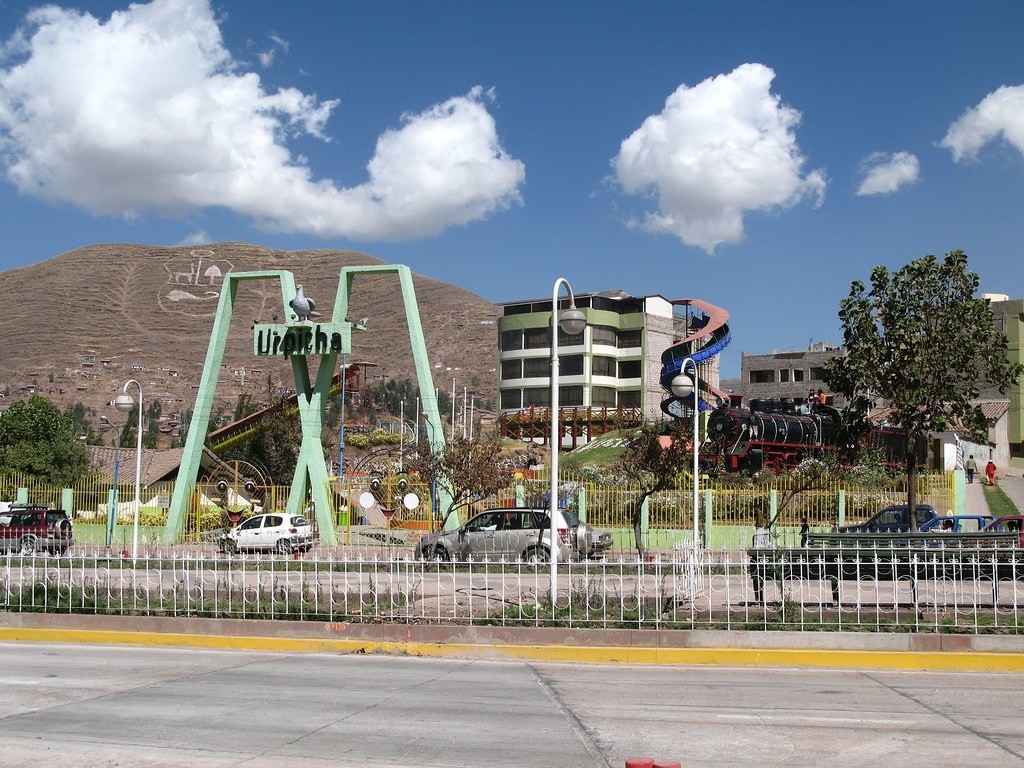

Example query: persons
[799,518,809,548]
[526,443,537,470]
[966,455,978,483]
[805,389,826,414]
[931,520,952,532]
[1006,521,1018,532]
[986,460,996,486]
[924,512,933,522]
[492,516,517,530]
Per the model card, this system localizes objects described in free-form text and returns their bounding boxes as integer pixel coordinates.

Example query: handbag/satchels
[966,473,968,478]
[985,477,990,482]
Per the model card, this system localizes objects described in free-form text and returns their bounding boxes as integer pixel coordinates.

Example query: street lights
[548,278,588,600]
[669,357,696,567]
[115,377,142,569]
[100,415,120,490]
[340,354,350,479]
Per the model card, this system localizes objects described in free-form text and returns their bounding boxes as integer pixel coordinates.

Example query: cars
[979,515,1024,547]
[218,511,312,553]
[830,504,942,532]
[590,529,613,556]
[910,516,1000,532]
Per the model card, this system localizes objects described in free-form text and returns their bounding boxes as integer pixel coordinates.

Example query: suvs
[417,506,594,573]
[0,507,73,556]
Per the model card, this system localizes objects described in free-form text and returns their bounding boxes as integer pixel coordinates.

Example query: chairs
[900,513,906,523]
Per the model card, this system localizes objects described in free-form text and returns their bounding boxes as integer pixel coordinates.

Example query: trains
[696,396,928,475]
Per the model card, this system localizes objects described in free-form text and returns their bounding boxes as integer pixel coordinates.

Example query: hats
[988,460,992,463]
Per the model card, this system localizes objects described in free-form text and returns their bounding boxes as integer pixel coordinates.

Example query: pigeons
[289,284,321,322]
